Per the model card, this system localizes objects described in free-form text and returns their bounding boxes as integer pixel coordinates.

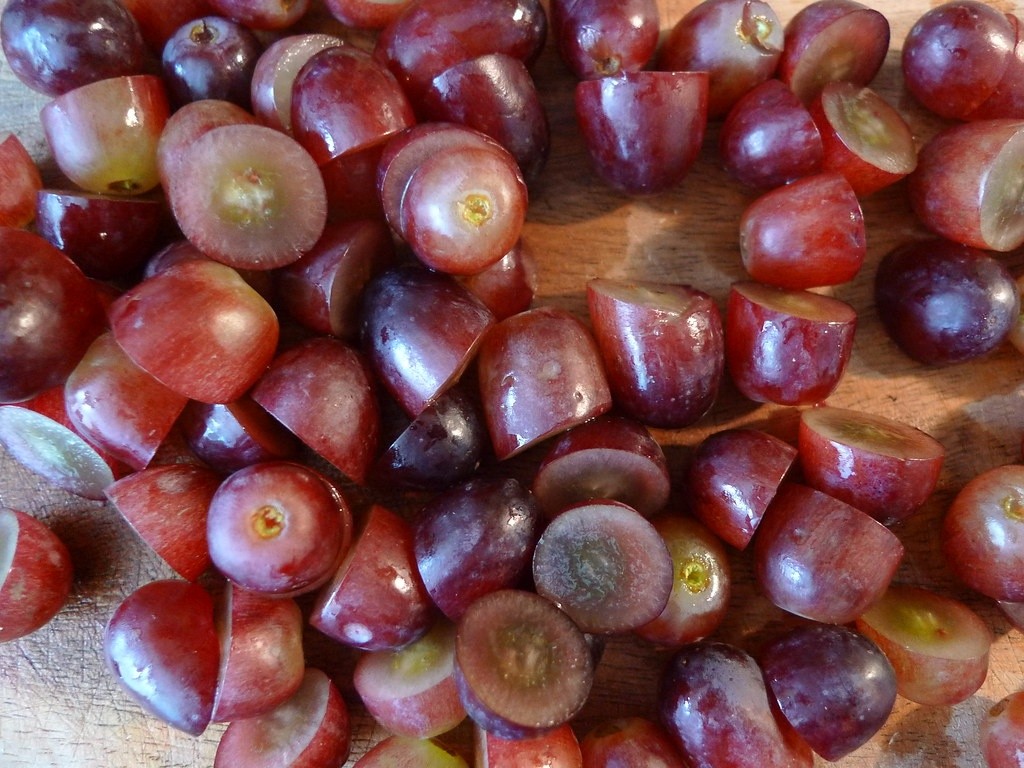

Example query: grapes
[0,0,1024,768]
